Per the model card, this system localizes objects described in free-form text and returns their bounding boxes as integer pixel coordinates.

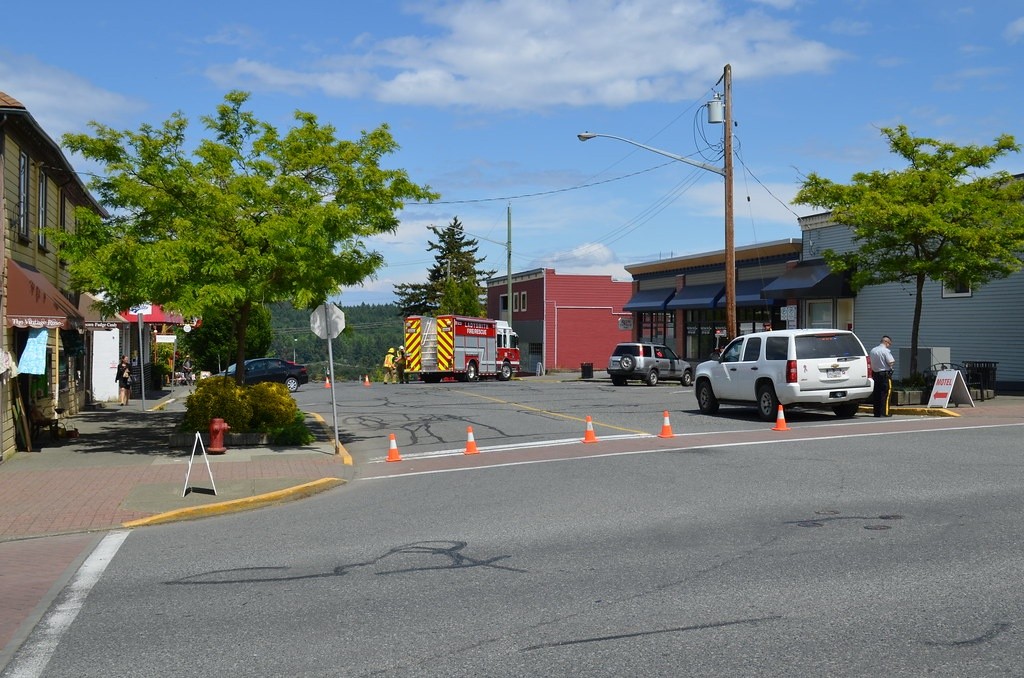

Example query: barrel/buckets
[54,423,66,437]
[65,421,75,437]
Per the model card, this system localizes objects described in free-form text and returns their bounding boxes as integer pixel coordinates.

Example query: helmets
[387,347,394,353]
[399,345,405,351]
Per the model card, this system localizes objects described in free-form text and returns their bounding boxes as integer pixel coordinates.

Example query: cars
[212,358,308,392]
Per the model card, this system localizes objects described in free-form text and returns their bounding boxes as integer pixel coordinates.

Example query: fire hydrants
[207,418,231,453]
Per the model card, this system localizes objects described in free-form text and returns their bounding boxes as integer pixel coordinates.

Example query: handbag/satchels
[127,374,137,385]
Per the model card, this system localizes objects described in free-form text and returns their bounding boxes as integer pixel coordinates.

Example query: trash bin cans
[581,362,593,379]
[963,360,999,397]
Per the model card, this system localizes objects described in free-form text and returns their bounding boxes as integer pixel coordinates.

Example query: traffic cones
[772,405,791,431]
[364,375,370,386]
[325,377,331,388]
[657,411,675,438]
[581,416,599,443]
[464,426,480,455]
[386,433,402,461]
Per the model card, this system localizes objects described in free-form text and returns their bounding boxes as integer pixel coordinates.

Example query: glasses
[885,340,892,346]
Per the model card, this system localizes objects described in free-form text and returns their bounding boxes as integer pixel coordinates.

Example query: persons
[870,336,896,417]
[115,354,134,406]
[183,355,194,386]
[762,322,772,332]
[383,346,409,384]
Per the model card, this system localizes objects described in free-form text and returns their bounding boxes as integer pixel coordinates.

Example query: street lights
[578,131,736,340]
[293,337,297,363]
[427,224,512,327]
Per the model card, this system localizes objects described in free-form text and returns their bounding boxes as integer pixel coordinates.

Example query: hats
[185,355,189,357]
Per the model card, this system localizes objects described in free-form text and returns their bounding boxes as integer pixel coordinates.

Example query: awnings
[667,277,787,309]
[623,288,676,311]
[78,291,129,328]
[723,305,786,324]
[760,254,858,299]
[6,258,85,330]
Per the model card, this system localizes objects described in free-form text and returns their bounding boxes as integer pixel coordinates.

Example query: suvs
[694,329,874,420]
[607,342,693,387]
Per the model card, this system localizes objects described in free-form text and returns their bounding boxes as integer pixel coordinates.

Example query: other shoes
[120,402,124,406]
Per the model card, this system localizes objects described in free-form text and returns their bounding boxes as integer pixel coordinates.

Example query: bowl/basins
[55,409,65,414]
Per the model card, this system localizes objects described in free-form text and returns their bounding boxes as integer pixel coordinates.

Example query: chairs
[26,404,59,441]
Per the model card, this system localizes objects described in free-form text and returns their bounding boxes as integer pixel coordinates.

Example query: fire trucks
[403,315,520,383]
